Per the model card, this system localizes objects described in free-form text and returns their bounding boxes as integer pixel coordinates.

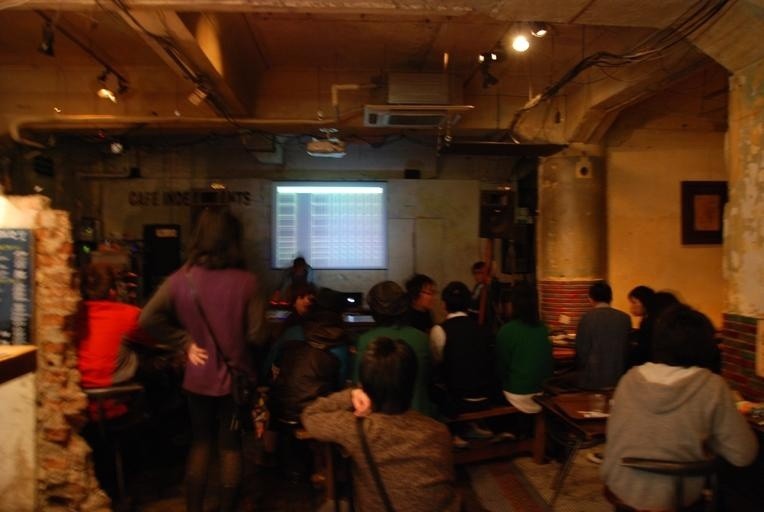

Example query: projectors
[306,139,347,158]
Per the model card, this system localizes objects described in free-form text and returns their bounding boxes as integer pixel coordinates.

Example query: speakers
[480,189,515,239]
[143,223,181,298]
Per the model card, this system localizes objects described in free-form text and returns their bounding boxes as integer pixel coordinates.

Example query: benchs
[443,404,545,500]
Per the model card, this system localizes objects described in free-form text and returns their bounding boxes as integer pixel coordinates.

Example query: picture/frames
[681,179,729,245]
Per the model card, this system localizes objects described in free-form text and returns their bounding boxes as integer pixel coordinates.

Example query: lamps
[34,9,129,104]
[464,21,549,89]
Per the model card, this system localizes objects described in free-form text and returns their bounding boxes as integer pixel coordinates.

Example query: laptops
[343,292,362,307]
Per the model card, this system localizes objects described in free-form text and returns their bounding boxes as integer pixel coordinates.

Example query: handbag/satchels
[232,373,259,408]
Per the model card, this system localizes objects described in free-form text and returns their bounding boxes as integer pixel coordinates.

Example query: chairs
[295,431,351,511]
[82,384,144,501]
[531,395,607,505]
[619,450,717,512]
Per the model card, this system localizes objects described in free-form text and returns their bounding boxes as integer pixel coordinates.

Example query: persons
[76,241,161,510]
[302,337,461,510]
[603,308,758,510]
[140,206,273,510]
[258,256,686,448]
